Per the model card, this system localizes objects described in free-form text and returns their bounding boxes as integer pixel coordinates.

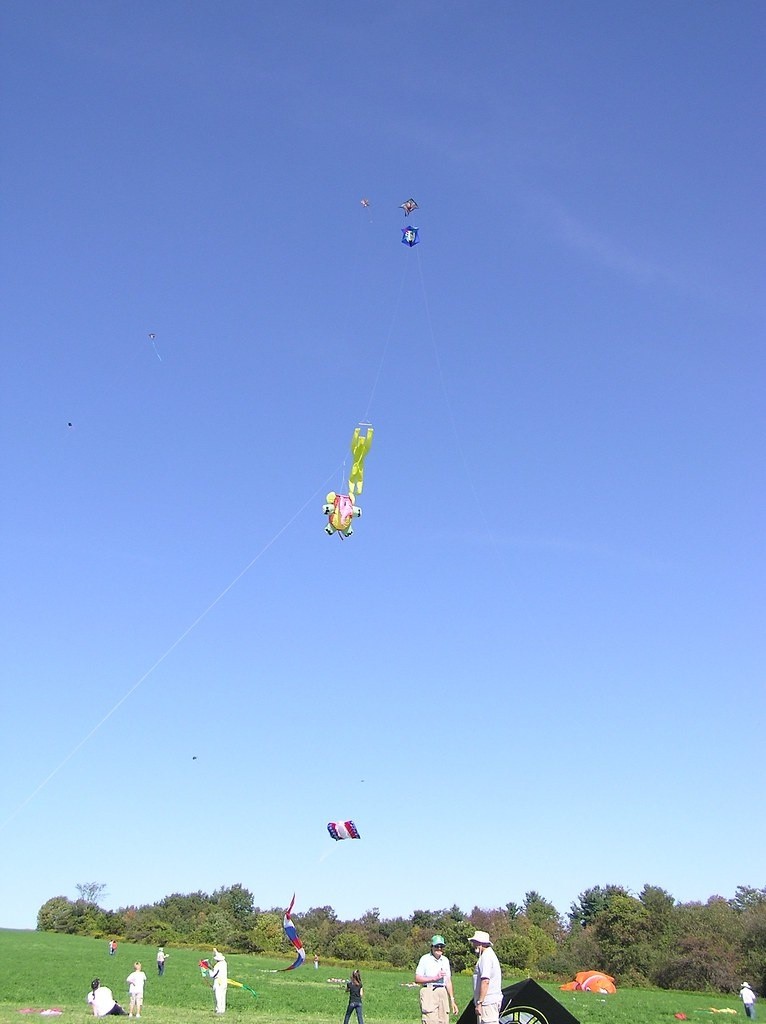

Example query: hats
[741,982,751,988]
[213,953,225,961]
[468,931,493,947]
[431,935,446,946]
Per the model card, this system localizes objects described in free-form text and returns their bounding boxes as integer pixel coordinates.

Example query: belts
[423,985,444,991]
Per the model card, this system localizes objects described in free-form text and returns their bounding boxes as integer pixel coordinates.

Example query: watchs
[477,1000,482,1005]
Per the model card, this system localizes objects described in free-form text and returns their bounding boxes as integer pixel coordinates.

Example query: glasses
[434,945,445,948]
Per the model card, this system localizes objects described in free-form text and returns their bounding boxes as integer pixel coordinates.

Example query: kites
[401,225,419,247]
[328,820,360,841]
[198,958,258,998]
[149,333,162,364]
[361,199,368,208]
[262,894,306,976]
[321,491,360,541]
[398,198,420,217]
[349,427,374,505]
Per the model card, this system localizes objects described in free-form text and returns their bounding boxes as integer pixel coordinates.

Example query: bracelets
[449,997,455,1000]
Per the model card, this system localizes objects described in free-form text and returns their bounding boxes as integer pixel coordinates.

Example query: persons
[343,970,364,1024]
[157,948,170,975]
[414,935,459,1024]
[313,955,319,968]
[467,931,503,1024]
[108,939,117,955]
[212,948,227,1013]
[126,962,147,1017]
[87,979,128,1016]
[739,982,756,1020]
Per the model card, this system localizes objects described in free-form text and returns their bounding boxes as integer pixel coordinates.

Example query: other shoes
[129,1013,133,1017]
[136,1014,140,1017]
